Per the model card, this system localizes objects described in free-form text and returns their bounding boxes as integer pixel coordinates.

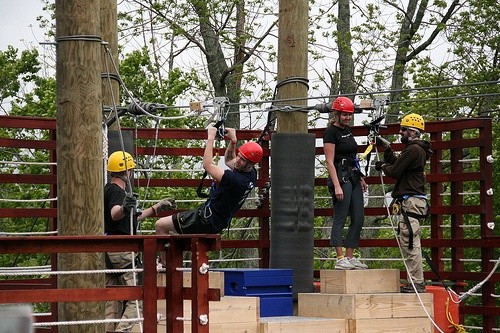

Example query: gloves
[375,161,384,171]
[384,148,395,165]
[152,198,176,216]
[122,193,139,216]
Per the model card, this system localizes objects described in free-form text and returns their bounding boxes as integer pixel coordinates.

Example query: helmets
[331,97,354,113]
[238,141,263,164]
[400,113,425,131]
[106,150,135,172]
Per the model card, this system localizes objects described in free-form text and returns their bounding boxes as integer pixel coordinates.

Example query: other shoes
[155,257,167,270]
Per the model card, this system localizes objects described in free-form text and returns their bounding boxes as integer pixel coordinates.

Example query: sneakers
[335,256,356,270]
[348,256,368,269]
[403,282,426,292]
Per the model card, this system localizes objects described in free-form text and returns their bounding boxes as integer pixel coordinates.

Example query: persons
[368,113,431,293]
[154,126,263,268]
[323,96,368,270]
[104,151,177,333]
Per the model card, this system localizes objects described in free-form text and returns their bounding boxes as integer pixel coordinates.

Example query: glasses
[399,126,415,132]
